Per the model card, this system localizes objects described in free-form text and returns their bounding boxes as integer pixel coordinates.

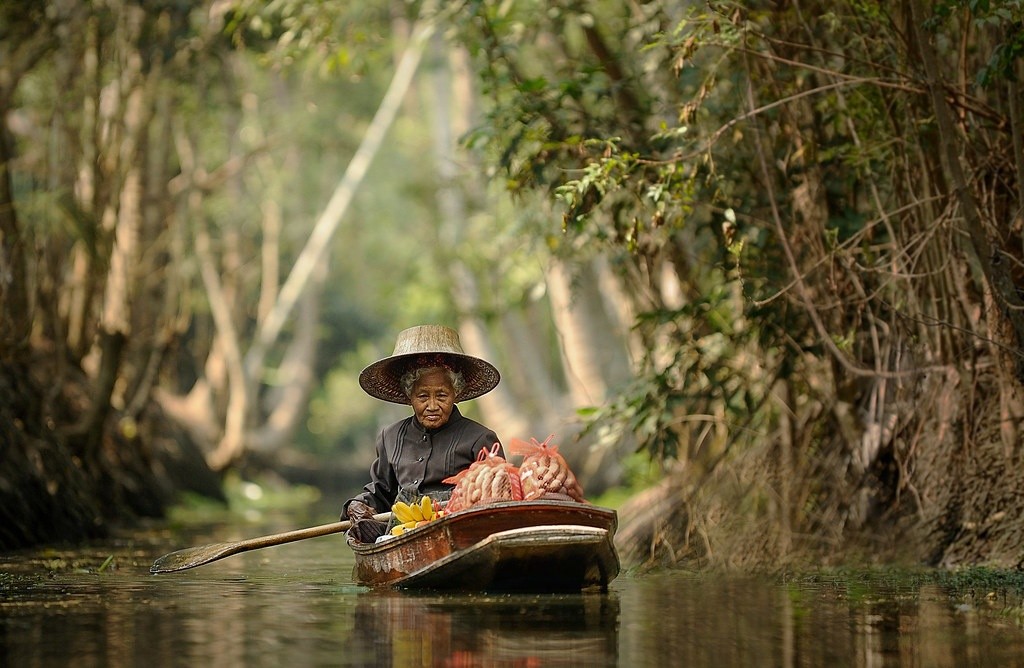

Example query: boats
[342,499,621,590]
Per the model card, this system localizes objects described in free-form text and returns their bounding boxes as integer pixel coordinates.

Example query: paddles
[149,500,451,573]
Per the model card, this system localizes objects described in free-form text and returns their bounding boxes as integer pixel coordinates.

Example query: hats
[358,325,501,406]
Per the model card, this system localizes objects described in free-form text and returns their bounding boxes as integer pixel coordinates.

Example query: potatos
[448,455,582,512]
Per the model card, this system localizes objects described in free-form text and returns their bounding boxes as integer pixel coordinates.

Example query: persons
[338,356,508,544]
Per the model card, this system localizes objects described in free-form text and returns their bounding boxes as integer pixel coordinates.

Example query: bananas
[391,496,444,534]
